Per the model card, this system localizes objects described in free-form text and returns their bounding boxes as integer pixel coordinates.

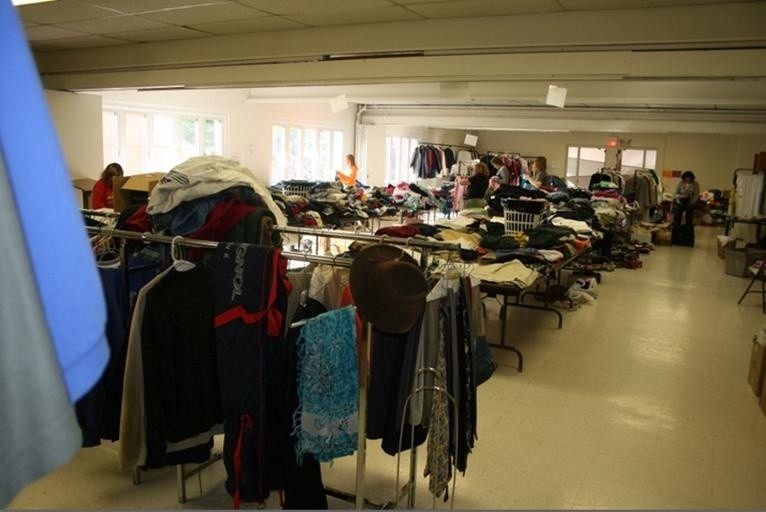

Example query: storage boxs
[721,246,765,277]
[110,170,171,214]
[746,332,764,400]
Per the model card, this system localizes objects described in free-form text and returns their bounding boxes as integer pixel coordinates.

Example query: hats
[347,240,425,337]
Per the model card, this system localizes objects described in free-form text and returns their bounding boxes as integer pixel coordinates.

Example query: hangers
[418,141,451,152]
[83,216,465,324]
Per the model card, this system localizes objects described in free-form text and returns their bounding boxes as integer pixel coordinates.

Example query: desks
[291,216,378,258]
[377,201,440,230]
[466,238,600,373]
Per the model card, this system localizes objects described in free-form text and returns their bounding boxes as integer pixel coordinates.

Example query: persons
[670,171,700,224]
[89,162,123,212]
[457,154,548,201]
[335,152,358,184]
